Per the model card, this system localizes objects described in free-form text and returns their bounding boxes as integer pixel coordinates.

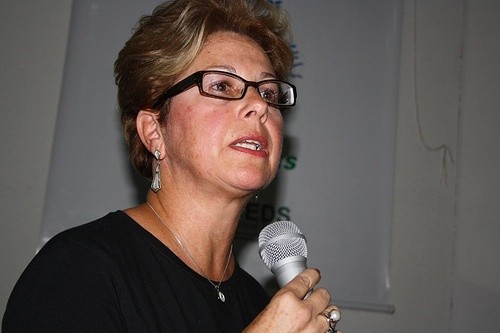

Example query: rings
[319,307,340,332]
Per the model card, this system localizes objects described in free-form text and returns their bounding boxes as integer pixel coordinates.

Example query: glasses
[148,68,299,112]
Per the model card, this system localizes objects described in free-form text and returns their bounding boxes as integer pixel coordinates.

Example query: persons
[3,0,340,332]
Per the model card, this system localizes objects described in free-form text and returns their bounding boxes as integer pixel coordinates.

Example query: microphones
[258,220,315,301]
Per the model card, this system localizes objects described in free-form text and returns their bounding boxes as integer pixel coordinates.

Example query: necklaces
[145,200,234,303]
